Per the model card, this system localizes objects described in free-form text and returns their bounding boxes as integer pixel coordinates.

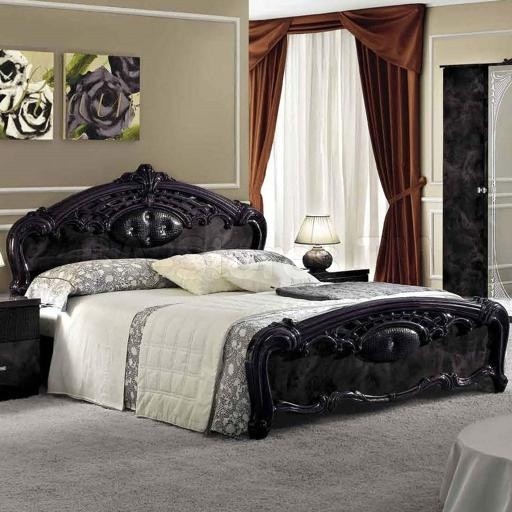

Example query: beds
[4,162,508,442]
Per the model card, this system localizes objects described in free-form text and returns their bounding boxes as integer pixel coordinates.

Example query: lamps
[293,214,341,272]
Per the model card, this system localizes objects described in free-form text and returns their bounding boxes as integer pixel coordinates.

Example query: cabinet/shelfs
[437,56,512,302]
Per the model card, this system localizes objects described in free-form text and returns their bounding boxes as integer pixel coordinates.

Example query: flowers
[0,48,53,141]
[62,54,142,140]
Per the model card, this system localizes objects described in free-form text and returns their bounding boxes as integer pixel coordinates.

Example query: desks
[438,415,512,511]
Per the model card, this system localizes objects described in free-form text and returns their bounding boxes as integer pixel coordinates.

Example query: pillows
[152,252,244,295]
[203,247,309,271]
[225,259,320,293]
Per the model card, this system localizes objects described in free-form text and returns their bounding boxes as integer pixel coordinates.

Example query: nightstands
[1,296,48,401]
[305,268,370,283]
